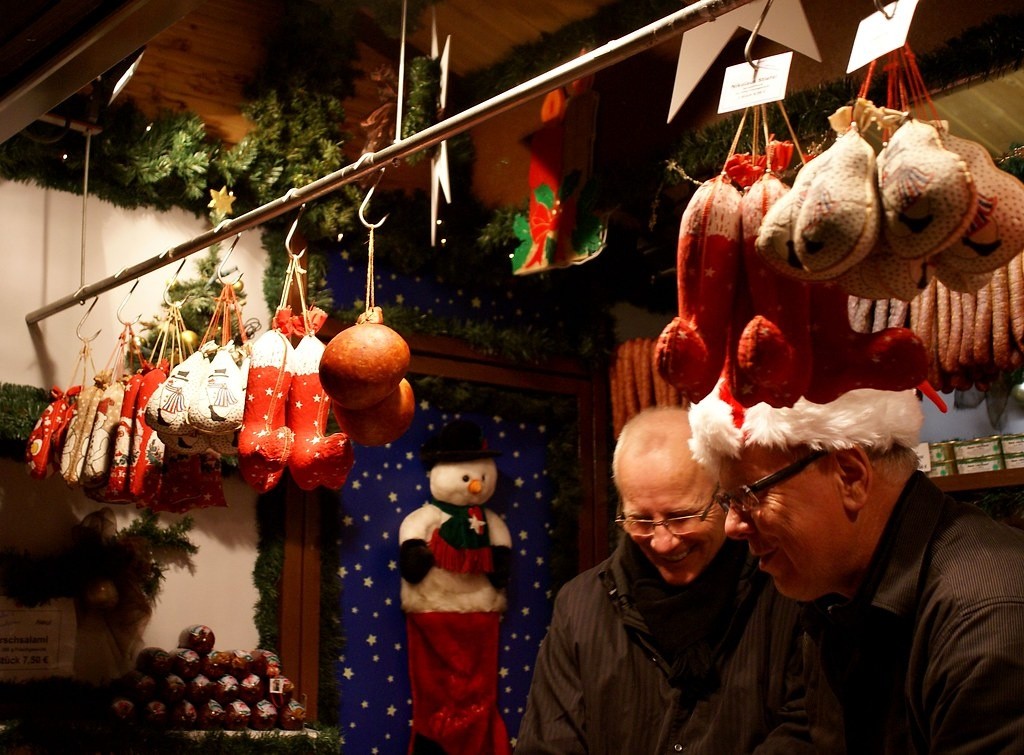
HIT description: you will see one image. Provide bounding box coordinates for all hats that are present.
[688,351,924,468]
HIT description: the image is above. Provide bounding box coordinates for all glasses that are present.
[614,486,721,537]
[714,446,828,514]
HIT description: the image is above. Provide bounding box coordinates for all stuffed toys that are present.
[396,427,514,755]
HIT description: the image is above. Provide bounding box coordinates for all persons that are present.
[688,365,1024,755]
[513,406,852,754]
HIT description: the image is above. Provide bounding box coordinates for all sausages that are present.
[108,626,307,732]
[607,335,697,441]
[846,251,1024,401]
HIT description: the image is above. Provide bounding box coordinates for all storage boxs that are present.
[0,595,154,682]
